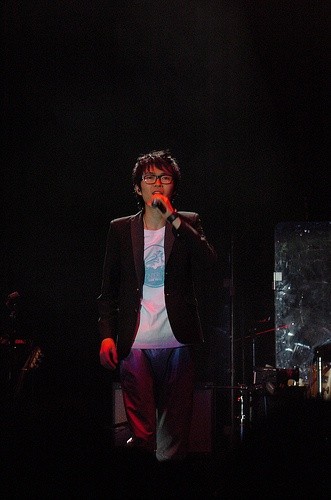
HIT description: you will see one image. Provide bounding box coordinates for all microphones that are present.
[152,199,161,208]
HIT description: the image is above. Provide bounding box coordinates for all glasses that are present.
[140,175,173,184]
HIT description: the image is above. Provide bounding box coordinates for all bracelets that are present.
[166,211,179,224]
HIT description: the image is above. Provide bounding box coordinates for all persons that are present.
[97,150,218,500]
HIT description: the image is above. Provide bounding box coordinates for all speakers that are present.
[112,382,218,459]
